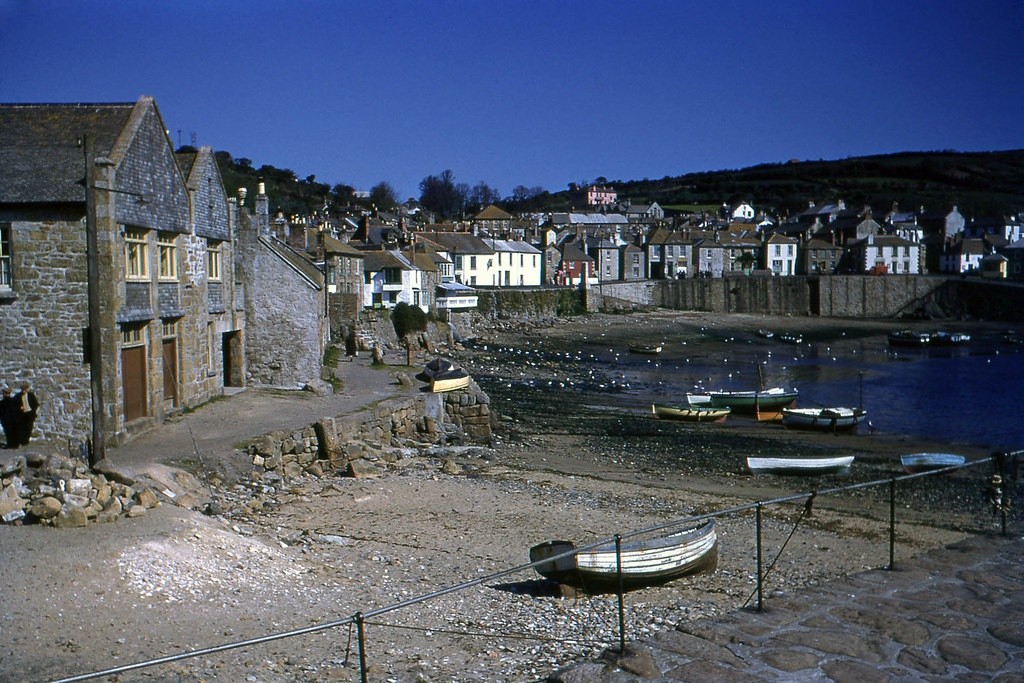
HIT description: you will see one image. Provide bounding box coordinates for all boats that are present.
[530,519,720,587]
[650,400,731,424]
[744,453,858,477]
[884,328,976,349]
[686,385,800,414]
[901,453,971,475]
[628,341,663,354]
[428,367,471,394]
[781,408,870,428]
[423,358,453,378]
[756,327,774,338]
[779,335,804,345]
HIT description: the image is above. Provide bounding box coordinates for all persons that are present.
[13,382,40,446]
[344,335,359,362]
[0,388,21,449]
